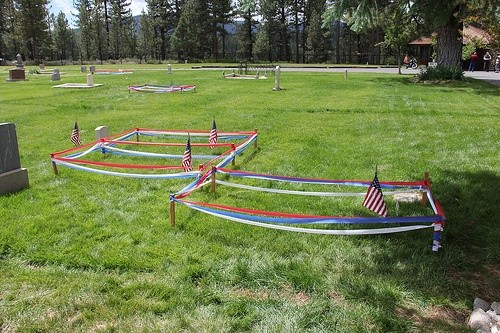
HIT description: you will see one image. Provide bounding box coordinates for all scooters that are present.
[403,55,419,69]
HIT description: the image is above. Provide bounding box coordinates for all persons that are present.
[403,51,410,69]
[468,51,478,72]
[493,50,500,73]
[483,50,492,72]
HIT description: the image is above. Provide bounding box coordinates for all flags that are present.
[69,121,81,146]
[181,136,192,173]
[362,171,388,218]
[208,119,218,150]
